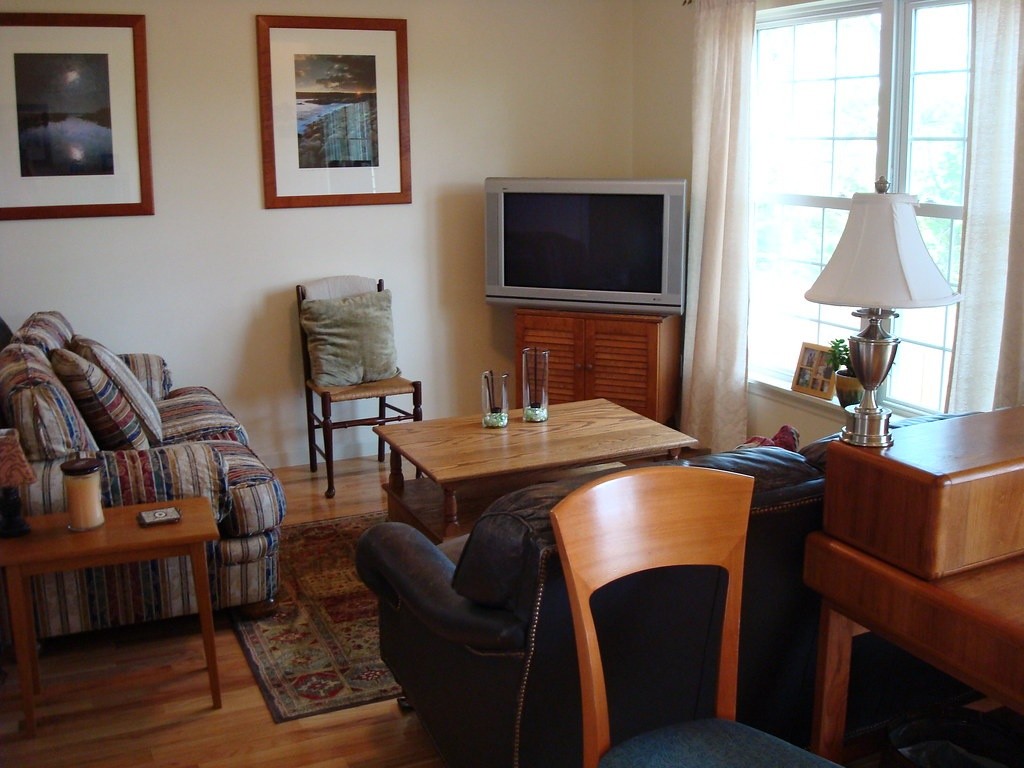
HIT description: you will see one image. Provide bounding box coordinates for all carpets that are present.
[226,508,410,724]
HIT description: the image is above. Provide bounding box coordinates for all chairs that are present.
[296,274,422,498]
[549,465,849,768]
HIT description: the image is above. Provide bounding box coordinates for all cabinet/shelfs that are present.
[803,405,1024,763]
[515,308,683,429]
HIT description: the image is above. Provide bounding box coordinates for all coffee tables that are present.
[373,397,699,548]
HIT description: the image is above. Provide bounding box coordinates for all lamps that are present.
[0,428,35,539]
[804,176,960,448]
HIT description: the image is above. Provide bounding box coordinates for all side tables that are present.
[0,497,222,738]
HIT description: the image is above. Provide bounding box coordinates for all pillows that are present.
[300,289,402,388]
[51,346,152,452]
[70,334,163,444]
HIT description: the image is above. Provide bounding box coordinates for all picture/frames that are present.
[791,341,839,401]
[0,12,156,222]
[254,14,412,210]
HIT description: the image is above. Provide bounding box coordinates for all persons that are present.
[806,353,817,367]
[823,364,832,379]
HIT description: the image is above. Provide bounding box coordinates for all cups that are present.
[482,370,508,427]
[522,346,549,422]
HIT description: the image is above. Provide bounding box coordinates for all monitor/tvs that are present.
[484,177,687,316]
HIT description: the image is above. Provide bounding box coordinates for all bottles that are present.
[60,458,105,530]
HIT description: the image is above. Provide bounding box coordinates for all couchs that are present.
[0,310,286,645]
[354,411,986,768]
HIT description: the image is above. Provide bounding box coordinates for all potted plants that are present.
[826,338,866,409]
